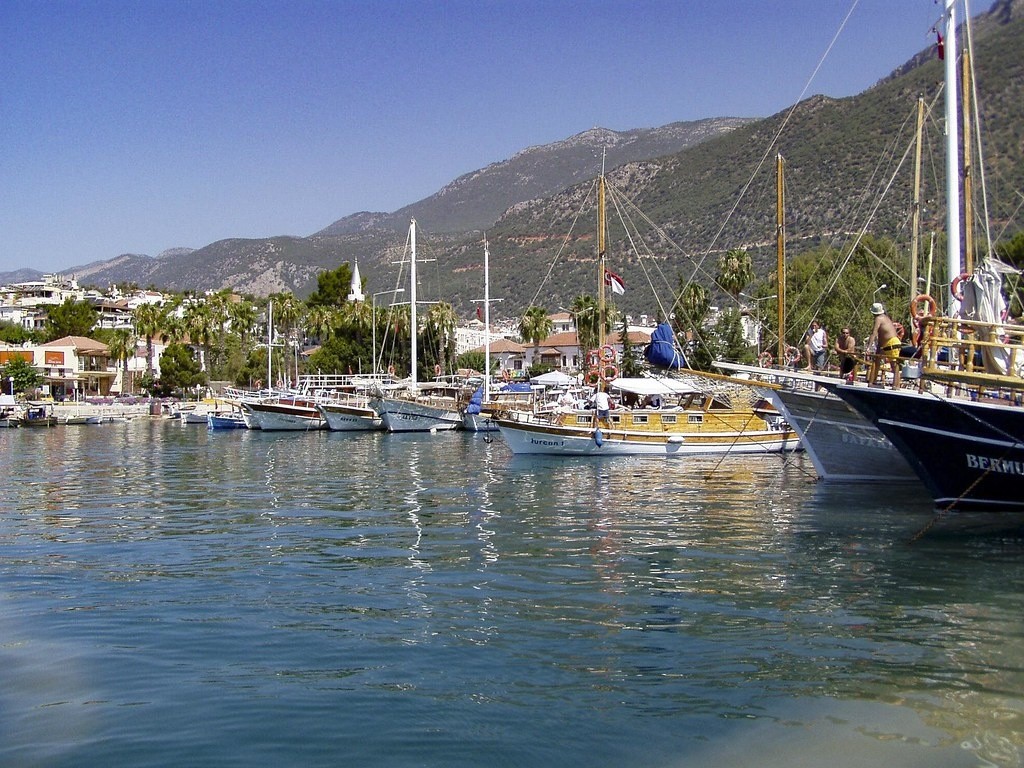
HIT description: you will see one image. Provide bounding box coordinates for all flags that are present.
[611,277,625,296]
[604,268,624,290]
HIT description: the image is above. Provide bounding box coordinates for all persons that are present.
[865,303,902,390]
[588,387,663,428]
[555,389,573,425]
[834,327,857,379]
[804,320,829,372]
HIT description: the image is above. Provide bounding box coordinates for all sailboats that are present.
[0,0,1024,519]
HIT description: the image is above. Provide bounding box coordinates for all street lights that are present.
[739,292,778,357]
[872,283,887,303]
[371,288,406,374]
[917,276,948,317]
[559,306,594,369]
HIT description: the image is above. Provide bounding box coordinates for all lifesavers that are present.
[586,350,602,368]
[502,370,508,380]
[893,318,940,339]
[388,366,395,376]
[586,371,601,388]
[953,309,977,334]
[951,273,972,302]
[994,301,1011,345]
[599,344,616,362]
[785,346,800,363]
[758,351,772,366]
[911,294,936,318]
[435,365,441,375]
[599,364,618,382]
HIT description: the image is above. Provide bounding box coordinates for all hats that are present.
[869,303,884,315]
[563,386,568,390]
